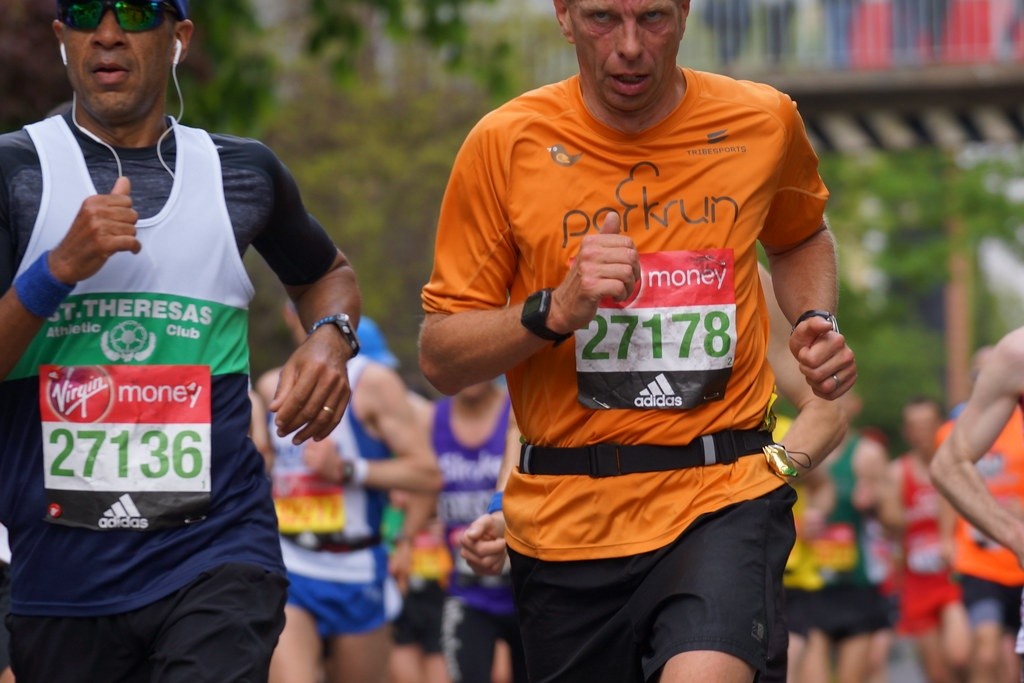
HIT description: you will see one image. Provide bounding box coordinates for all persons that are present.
[419,0,859,683]
[248,301,523,683]
[0,0,360,683]
[459,261,1024,683]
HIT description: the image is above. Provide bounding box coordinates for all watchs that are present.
[521,288,575,348]
[790,310,838,334]
[309,313,360,358]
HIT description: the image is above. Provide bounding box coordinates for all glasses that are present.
[57,0,176,33]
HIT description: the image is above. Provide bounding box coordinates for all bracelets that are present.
[488,492,504,513]
[15,251,75,318]
[354,459,367,484]
[339,458,352,484]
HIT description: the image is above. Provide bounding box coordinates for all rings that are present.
[832,376,840,386]
[323,406,334,414]
